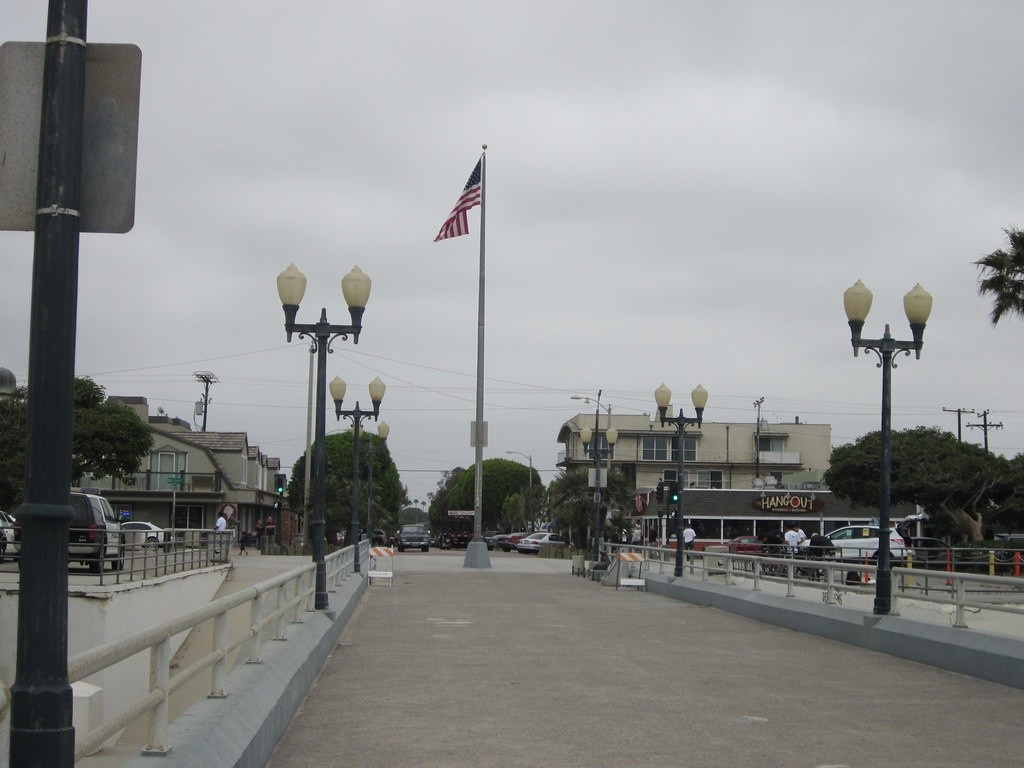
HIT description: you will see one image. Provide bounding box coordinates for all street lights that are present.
[577,408,619,559]
[841,278,935,614]
[571,396,616,468]
[350,420,391,546]
[507,451,534,490]
[325,372,386,573]
[275,262,374,614]
[654,381,709,575]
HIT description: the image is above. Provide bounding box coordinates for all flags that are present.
[434,158,482,243]
[636,495,643,512]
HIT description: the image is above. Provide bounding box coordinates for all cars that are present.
[517,532,575,554]
[702,535,792,555]
[0,512,22,554]
[334,526,388,547]
[910,536,958,560]
[119,521,174,550]
[485,529,531,554]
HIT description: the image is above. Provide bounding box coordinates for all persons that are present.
[648,525,656,559]
[621,523,641,551]
[214,511,226,530]
[684,524,697,560]
[266,517,276,544]
[238,531,248,555]
[255,520,265,550]
[759,523,834,575]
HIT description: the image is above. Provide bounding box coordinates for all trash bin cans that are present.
[705,546,730,576]
[185,532,200,547]
[572,555,585,577]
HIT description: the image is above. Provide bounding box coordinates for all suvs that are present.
[797,524,908,566]
[396,524,430,550]
[13,485,138,572]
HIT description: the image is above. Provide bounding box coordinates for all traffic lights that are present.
[277,477,283,496]
[670,483,680,505]
[669,509,679,519]
[655,483,664,504]
